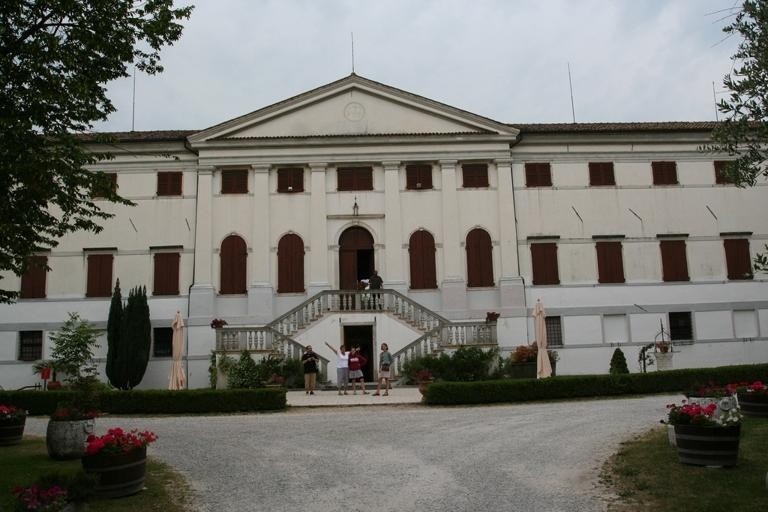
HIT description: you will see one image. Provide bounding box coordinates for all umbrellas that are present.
[168,310,186,390]
[532,298,552,379]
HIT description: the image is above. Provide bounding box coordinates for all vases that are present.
[417,380,433,401]
[91,447,147,497]
[0,418,26,444]
[47,417,96,461]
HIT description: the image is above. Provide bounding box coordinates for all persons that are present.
[301,345,319,395]
[371,342,394,396]
[370,270,383,310]
[348,347,369,394]
[324,341,360,395]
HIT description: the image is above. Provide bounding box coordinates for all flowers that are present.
[660,379,768,467]
[84,426,159,452]
[48,402,98,422]
[416,369,434,380]
[0,405,30,417]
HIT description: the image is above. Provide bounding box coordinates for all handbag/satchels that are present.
[382,364,389,371]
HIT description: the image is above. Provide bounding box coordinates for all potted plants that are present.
[29,358,55,379]
[45,360,67,390]
